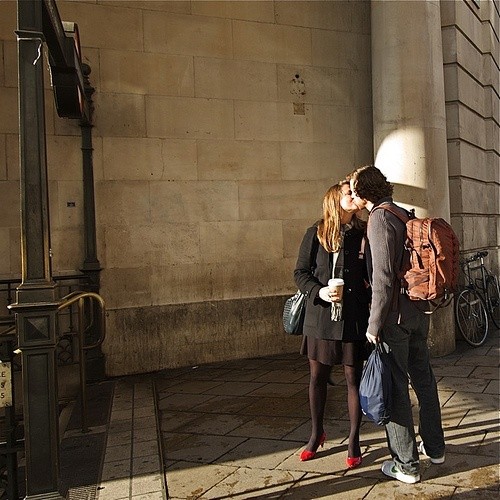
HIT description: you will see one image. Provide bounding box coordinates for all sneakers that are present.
[382,460,420,484]
[420,441,446,463]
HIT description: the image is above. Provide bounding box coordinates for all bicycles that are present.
[455,250,500,348]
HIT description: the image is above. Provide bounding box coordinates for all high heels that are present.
[301,431,326,461]
[346,456,362,468]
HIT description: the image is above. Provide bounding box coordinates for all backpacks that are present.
[371,204,460,301]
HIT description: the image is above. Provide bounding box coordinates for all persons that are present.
[293,180,369,468]
[345,166,445,483]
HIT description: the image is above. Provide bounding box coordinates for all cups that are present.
[328,279,344,301]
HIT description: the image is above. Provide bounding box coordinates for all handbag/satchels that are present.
[359,339,391,425]
[283,291,305,334]
